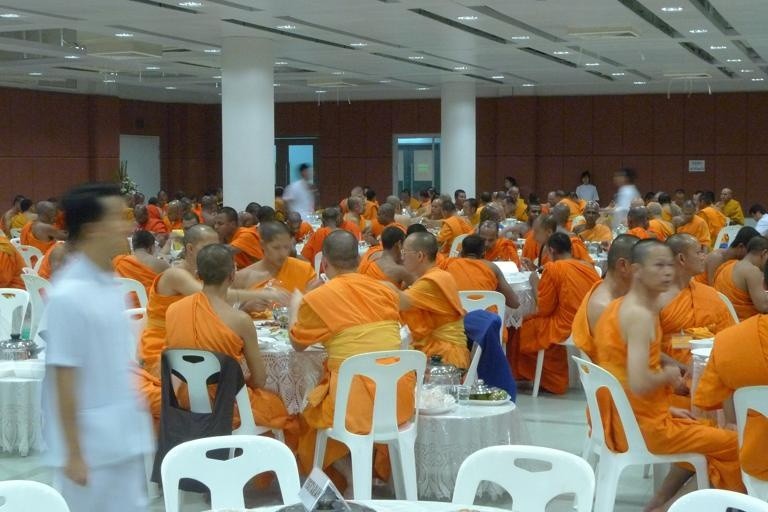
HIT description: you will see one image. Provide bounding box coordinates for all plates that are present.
[156,249,185,265]
[414,352,511,415]
[246,305,325,349]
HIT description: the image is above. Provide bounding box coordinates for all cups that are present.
[299,208,628,259]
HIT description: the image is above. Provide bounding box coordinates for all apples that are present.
[469,390,507,400]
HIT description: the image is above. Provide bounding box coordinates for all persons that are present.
[280,164,319,223]
[503,174,520,199]
[0,193,68,302]
[112,187,767,512]
[574,169,601,206]
[609,168,642,221]
[36,182,156,512]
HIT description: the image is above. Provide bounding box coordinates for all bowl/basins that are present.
[688,336,715,365]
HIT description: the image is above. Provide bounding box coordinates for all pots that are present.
[0,330,48,363]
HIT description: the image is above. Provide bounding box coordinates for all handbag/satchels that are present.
[151,347,245,492]
